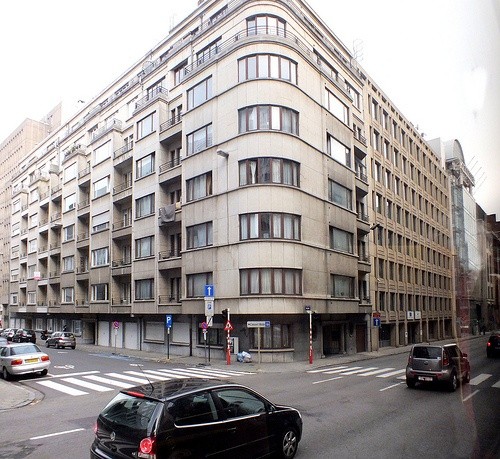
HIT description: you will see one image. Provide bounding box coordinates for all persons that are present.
[479,317,486,335]
[62,325,67,332]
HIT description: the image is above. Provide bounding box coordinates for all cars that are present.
[45,331,76,349]
[486,332,500,357]
[89,377,304,459]
[12,328,37,343]
[405,341,471,391]
[0,342,51,380]
[0,328,18,341]
